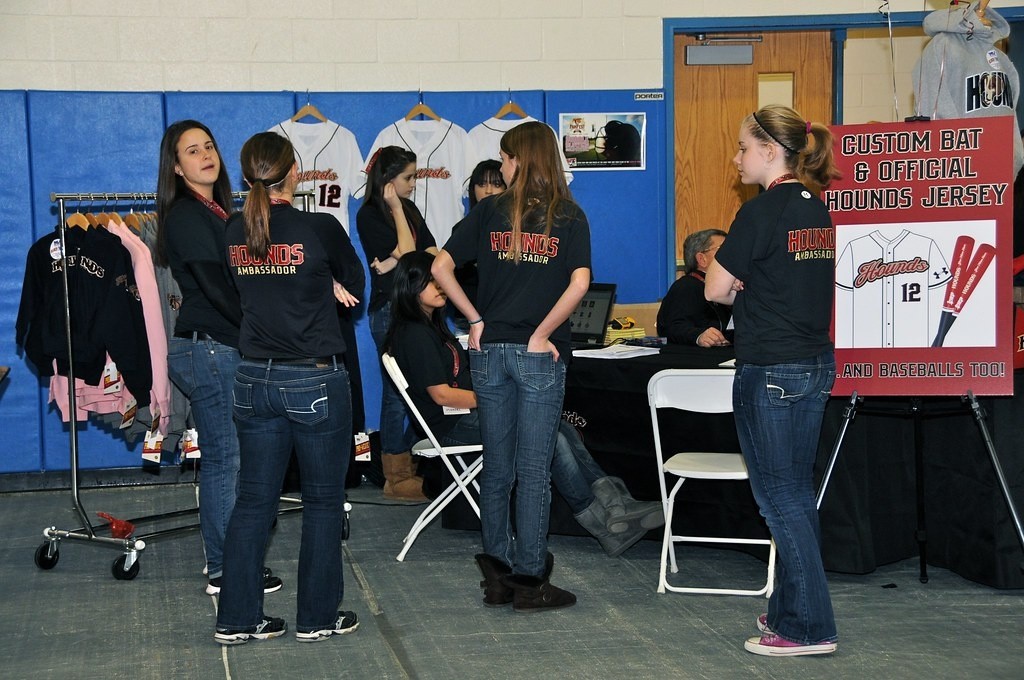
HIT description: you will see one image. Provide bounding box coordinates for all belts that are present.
[174,330,213,340]
[263,357,335,366]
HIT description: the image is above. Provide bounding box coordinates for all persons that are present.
[155,121,284,596]
[430,121,593,614]
[914,0,1024,258]
[705,106,839,656]
[657,229,735,347]
[383,252,666,558]
[452,159,507,335]
[356,147,439,501]
[214,132,360,645]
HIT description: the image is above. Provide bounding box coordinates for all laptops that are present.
[571,282,616,349]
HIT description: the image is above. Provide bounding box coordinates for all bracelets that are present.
[468,318,483,324]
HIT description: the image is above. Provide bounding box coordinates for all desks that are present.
[560,352,773,563]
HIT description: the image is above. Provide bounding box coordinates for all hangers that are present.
[66,191,243,234]
[495,87,527,119]
[289,89,327,124]
[406,88,441,123]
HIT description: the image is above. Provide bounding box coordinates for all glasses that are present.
[702,245,720,254]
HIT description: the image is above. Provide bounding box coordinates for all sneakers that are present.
[744,633,839,656]
[756,613,775,634]
[203,565,272,577]
[296,611,360,642]
[214,616,286,645]
[206,572,282,596]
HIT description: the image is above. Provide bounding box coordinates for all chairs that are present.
[383,351,484,560]
[645,369,778,600]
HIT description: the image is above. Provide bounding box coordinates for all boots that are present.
[573,498,647,558]
[474,554,511,608]
[505,554,575,613]
[380,450,428,501]
[592,475,665,534]
